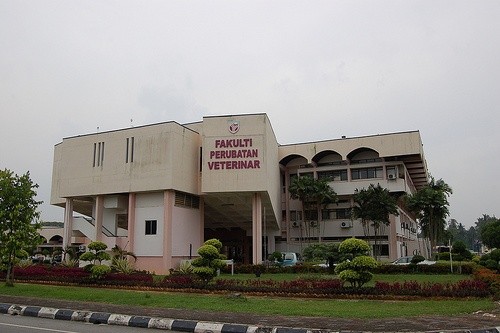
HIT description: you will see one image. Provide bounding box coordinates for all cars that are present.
[389,255,438,265]
[266,252,304,268]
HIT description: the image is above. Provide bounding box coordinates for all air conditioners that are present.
[388,173,395,179]
[340,220,352,228]
[292,221,301,227]
[310,220,317,226]
